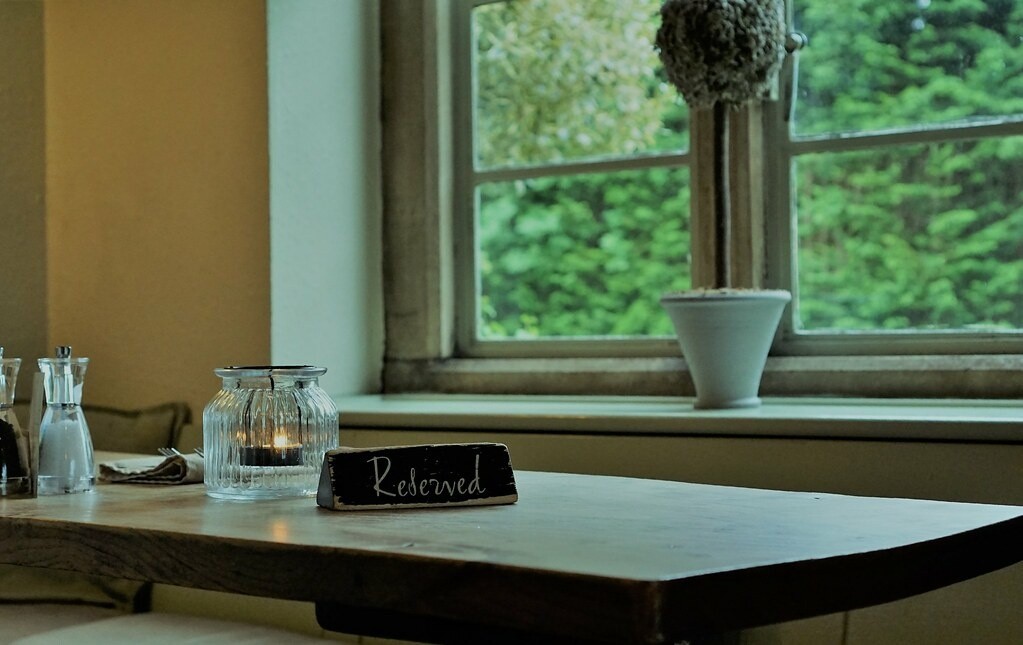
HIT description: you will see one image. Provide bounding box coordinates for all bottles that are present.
[37,346,96,496]
[203,364,339,500]
[0,346,37,499]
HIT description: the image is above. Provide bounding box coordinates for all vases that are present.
[659,289,792,409]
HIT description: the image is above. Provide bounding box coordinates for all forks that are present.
[157,446,183,459]
[194,447,203,458]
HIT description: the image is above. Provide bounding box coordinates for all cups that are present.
[658,289,791,409]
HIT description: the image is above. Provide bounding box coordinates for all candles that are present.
[239,430,303,466]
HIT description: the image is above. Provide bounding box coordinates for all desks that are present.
[1,447,1023,645]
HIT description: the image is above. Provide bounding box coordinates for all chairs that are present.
[0,400,194,614]
